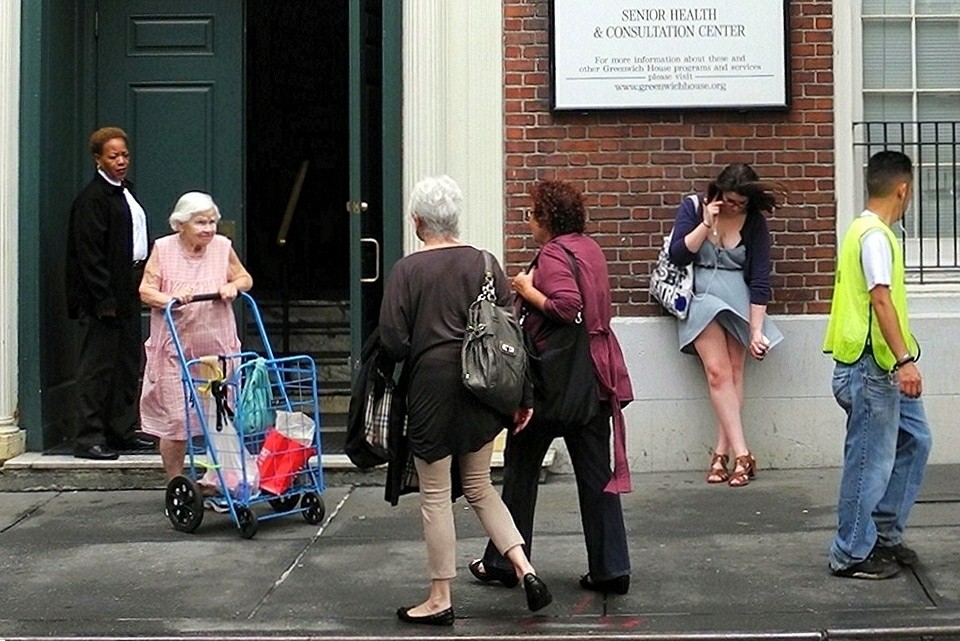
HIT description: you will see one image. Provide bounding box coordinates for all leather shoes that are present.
[74,443,119,460]
[128,438,156,448]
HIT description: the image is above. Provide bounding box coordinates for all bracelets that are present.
[897,354,914,367]
[704,220,711,228]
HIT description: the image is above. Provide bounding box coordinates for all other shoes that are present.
[202,493,229,513]
[396,605,454,628]
[523,573,554,613]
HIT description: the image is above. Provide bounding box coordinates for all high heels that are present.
[728,450,756,486]
[706,452,729,482]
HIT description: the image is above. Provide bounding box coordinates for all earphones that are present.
[713,228,718,236]
[901,192,906,202]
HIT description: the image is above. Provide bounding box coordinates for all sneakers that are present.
[872,544,918,565]
[829,554,901,579]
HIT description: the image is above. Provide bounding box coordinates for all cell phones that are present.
[714,190,723,202]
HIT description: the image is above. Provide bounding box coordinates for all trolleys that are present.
[165,289,326,540]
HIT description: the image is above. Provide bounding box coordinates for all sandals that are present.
[580,571,630,596]
[469,558,519,590]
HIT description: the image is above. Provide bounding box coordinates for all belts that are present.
[863,344,873,355]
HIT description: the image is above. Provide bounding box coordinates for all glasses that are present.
[721,193,749,209]
[528,210,538,216]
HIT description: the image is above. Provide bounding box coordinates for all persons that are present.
[469,180,634,594]
[66,127,156,460]
[822,151,933,579]
[668,164,789,486]
[380,177,552,625]
[138,192,253,512]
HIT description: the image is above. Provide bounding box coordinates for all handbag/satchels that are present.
[461,249,527,415]
[198,354,317,503]
[511,241,602,432]
[650,194,700,320]
